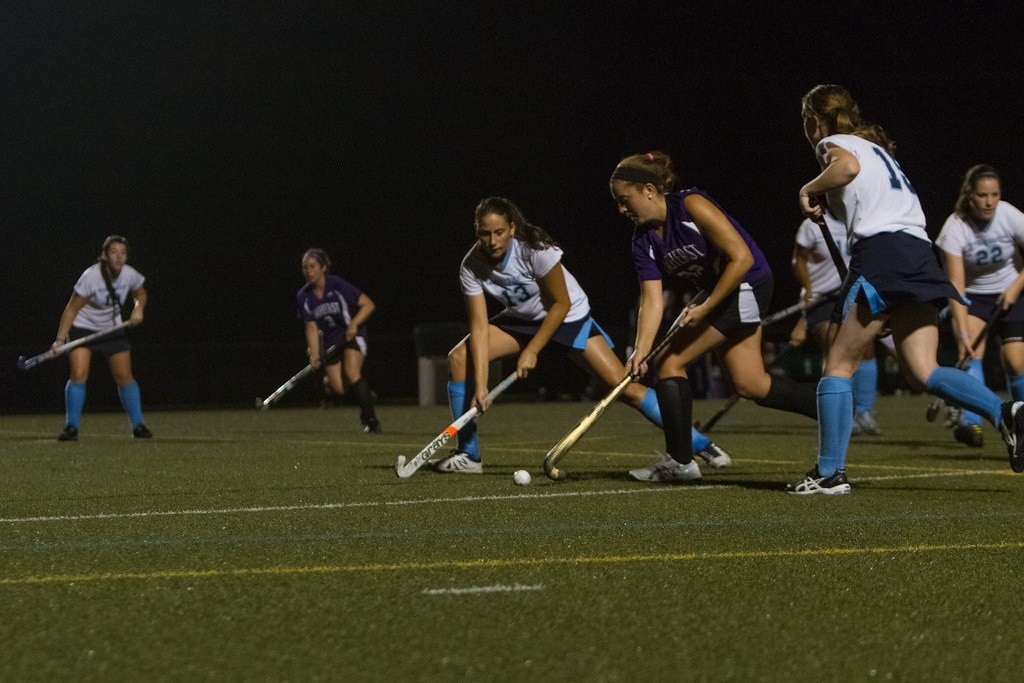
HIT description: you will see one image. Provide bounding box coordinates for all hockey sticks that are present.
[541,317,690,483]
[760,297,817,326]
[394,371,519,478]
[925,304,1004,423]
[692,340,797,434]
[255,338,348,411]
[17,319,130,372]
[808,195,891,339]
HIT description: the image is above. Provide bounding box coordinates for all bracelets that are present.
[57,337,65,343]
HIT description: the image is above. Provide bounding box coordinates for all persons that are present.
[935,163,1024,449]
[300,248,382,433]
[789,193,898,435]
[786,85,1024,494]
[610,153,857,481]
[49,235,153,441]
[428,196,731,473]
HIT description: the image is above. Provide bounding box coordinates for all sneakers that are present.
[628,453,702,482]
[786,462,851,495]
[698,442,731,467]
[133,424,152,439]
[427,448,483,473]
[945,405,959,427]
[995,399,1024,473]
[58,424,78,442]
[856,408,882,435]
[850,417,862,435]
[954,422,985,450]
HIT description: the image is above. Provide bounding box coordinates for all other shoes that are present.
[361,412,381,431]
[323,377,337,400]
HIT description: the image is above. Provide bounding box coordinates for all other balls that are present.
[513,469,531,486]
